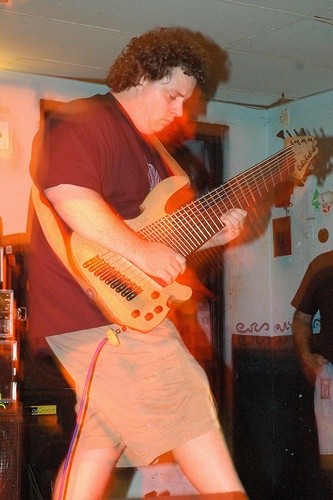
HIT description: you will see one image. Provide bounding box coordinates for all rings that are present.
[235,226,240,230]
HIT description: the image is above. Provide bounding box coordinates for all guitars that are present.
[65,127,320,334]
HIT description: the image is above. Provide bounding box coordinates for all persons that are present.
[25,26,249,500]
[291,250,333,500]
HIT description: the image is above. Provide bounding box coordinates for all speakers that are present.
[20,332,77,393]
[24,392,77,500]
[0,408,24,500]
